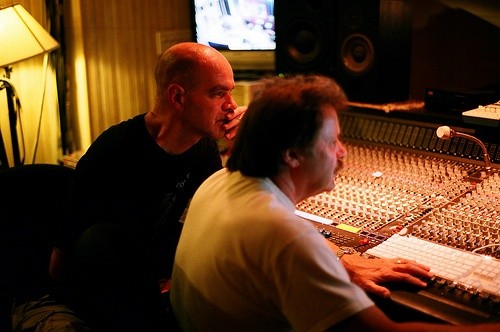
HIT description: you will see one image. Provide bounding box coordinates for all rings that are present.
[398,259,400,264]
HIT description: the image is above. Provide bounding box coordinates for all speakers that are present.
[275,0,412,103]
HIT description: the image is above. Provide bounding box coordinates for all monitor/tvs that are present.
[188,0,276,72]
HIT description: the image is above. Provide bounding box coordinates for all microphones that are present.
[435,126,491,170]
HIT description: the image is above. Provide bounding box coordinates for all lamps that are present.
[0,3,60,167]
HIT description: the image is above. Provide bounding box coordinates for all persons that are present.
[169,75,500,332]
[49,41,238,332]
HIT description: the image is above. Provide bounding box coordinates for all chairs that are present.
[0,163,75,332]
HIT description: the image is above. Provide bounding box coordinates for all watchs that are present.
[337,246,355,261]
